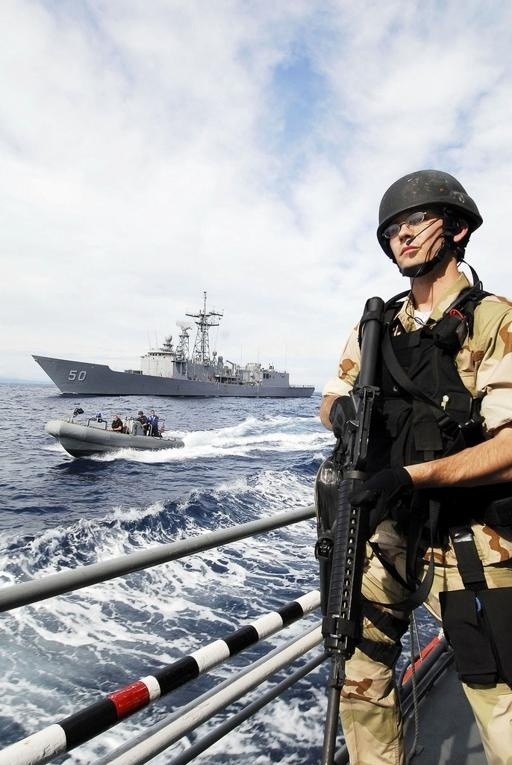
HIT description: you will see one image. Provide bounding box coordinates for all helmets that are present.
[377,170,483,260]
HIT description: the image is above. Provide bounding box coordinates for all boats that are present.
[31,291,315,399]
[45,408,184,458]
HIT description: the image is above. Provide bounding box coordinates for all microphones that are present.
[405,215,441,247]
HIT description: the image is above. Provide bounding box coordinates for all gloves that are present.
[349,467,415,542]
[329,396,357,437]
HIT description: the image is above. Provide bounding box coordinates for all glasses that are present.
[382,212,427,239]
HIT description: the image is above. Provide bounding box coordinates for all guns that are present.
[315,296,385,765]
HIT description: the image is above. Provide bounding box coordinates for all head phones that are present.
[441,212,465,239]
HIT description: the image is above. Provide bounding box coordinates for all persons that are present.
[148,409,159,437]
[137,411,149,435]
[318,167,512,765]
[107,415,123,433]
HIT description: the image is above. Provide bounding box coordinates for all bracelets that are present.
[113,428,114,430]
